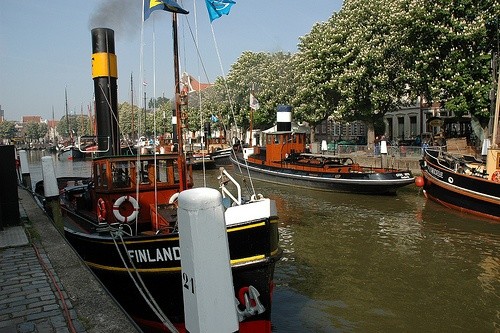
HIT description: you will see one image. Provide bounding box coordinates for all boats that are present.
[18,133,229,163]
[230,79,417,196]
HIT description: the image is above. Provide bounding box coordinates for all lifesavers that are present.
[96,198,106,221]
[113,195,140,223]
[491,171,500,182]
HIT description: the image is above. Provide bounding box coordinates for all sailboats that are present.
[35,1,283,333]
[418,50,499,220]
